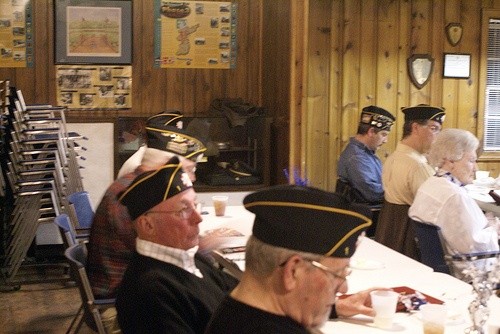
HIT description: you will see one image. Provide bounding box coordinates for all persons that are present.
[204,182,373,334]
[338,106,395,238]
[116,108,183,178]
[85,123,208,301]
[115,156,394,334]
[407,129,500,295]
[374,103,446,262]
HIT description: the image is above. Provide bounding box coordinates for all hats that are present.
[243,186,373,258]
[145,127,207,163]
[115,156,193,221]
[361,105,396,131]
[401,104,446,122]
[144,111,184,130]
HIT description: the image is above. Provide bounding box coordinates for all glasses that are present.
[301,257,353,284]
[144,202,201,221]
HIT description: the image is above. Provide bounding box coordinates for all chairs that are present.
[332,178,500,288]
[0,79,120,334]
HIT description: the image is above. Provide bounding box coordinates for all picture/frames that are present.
[53,0,134,65]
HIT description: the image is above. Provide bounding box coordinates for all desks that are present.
[464,180,500,216]
[186,189,500,334]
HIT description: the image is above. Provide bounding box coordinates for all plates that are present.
[472,179,495,186]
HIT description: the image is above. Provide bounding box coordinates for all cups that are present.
[476,170,489,182]
[212,196,229,216]
[419,305,448,334]
[369,290,400,330]
